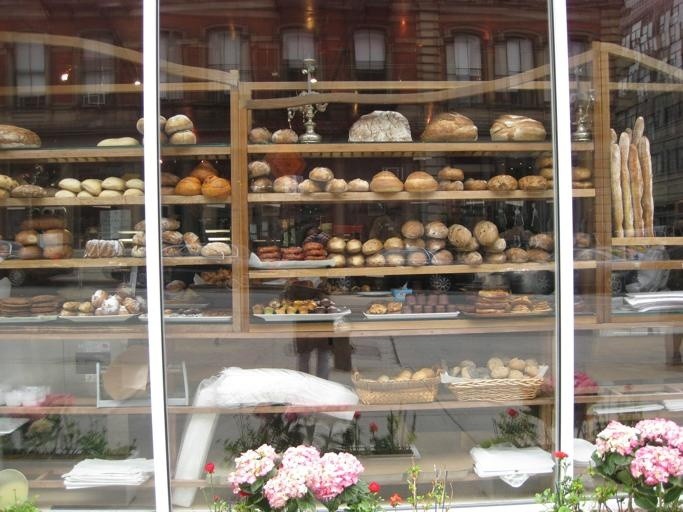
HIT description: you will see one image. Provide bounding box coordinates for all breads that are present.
[447,357,540,380]
[247,126,270,142]
[490,114,547,143]
[327,220,595,268]
[83,217,230,259]
[12,215,74,260]
[248,160,273,193]
[439,167,464,191]
[348,179,369,191]
[249,297,342,316]
[536,157,553,189]
[373,367,437,380]
[487,175,518,190]
[139,308,232,318]
[458,290,553,315]
[95,136,139,148]
[464,178,488,190]
[272,174,297,193]
[347,109,414,143]
[0,173,60,200]
[366,294,456,316]
[611,117,654,238]
[404,171,439,192]
[572,166,592,189]
[54,170,144,198]
[518,175,547,190]
[135,115,197,146]
[1,295,58,317]
[297,167,348,193]
[370,171,404,192]
[421,112,479,142]
[271,129,300,145]
[257,236,326,263]
[161,166,231,198]
[61,283,141,316]
[1,125,42,151]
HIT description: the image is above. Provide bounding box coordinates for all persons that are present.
[280,277,334,379]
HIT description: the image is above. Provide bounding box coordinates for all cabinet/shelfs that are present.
[1,30,238,488]
[604,42,682,434]
[238,41,604,491]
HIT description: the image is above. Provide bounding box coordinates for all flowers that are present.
[205,443,379,512]
[224,404,417,454]
[536,450,585,512]
[592,418,682,511]
[481,408,539,449]
[2,386,96,456]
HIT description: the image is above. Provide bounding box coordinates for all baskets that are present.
[444,367,543,401]
[351,365,441,405]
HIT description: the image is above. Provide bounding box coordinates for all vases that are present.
[333,446,421,484]
[1,453,139,507]
[470,442,557,479]
